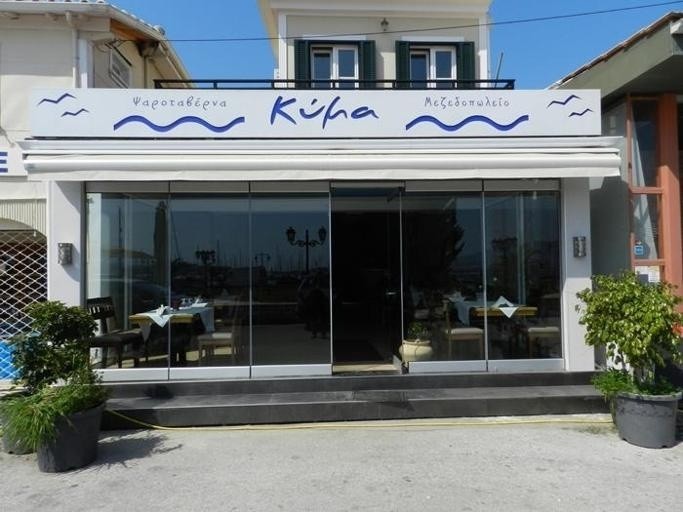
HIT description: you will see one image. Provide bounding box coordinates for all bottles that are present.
[634,239,647,260]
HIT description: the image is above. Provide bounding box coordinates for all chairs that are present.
[426,299,484,360]
[197,293,244,365]
[515,293,562,357]
[87,297,148,367]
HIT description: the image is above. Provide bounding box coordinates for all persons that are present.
[295,269,329,338]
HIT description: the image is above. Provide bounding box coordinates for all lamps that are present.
[380,18,389,31]
[574,236,587,258]
[58,243,72,264]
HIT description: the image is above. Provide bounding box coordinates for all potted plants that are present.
[399,322,434,367]
[0,300,114,473]
[575,271,683,448]
